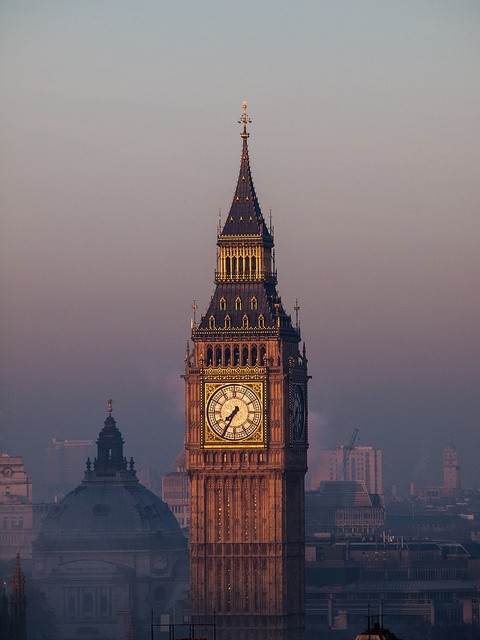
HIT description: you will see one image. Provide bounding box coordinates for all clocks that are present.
[286,351,310,451]
[199,356,271,452]
